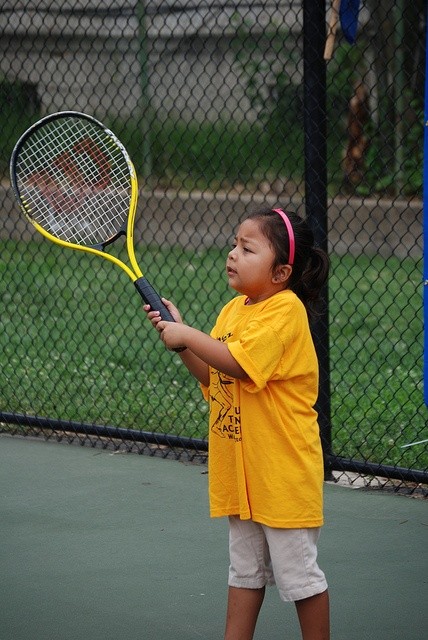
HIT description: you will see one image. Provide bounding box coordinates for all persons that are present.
[141,208,331,640]
